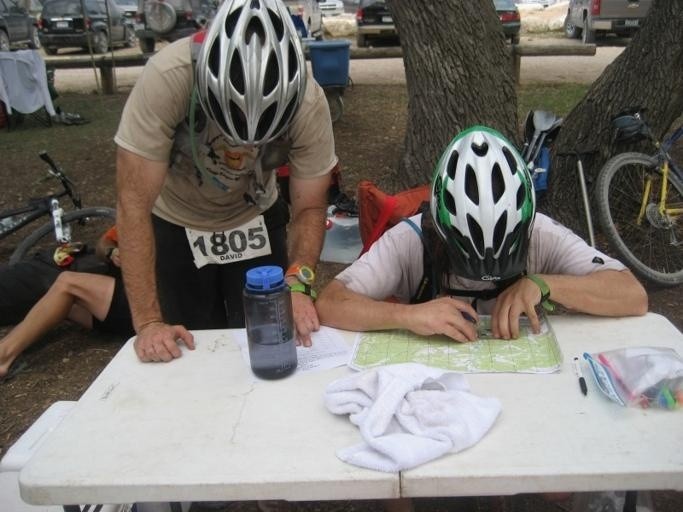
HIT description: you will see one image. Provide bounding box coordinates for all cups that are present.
[243,265,298,380]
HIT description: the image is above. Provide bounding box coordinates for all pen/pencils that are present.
[461,311,477,322]
[574,357,586,394]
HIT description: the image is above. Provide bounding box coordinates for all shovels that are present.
[521,109,563,171]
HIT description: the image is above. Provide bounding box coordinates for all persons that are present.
[0,266,136,381]
[1,224,120,328]
[112,0,340,363]
[313,125,649,343]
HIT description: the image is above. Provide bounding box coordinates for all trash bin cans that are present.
[308,40,350,87]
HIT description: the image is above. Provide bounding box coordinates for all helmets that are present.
[430,126,537,282]
[194,0,307,148]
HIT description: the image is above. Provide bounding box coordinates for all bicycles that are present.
[596,106,682,288]
[0,150,119,268]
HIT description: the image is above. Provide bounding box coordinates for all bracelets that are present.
[104,247,114,260]
[290,284,317,302]
[521,274,555,312]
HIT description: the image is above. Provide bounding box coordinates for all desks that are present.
[400,311,682,511]
[18,312,400,512]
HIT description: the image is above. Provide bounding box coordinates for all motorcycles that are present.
[320,85,344,123]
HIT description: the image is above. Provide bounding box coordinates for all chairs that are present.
[0,49,56,132]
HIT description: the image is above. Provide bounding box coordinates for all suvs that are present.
[136,0,218,54]
[38,0,137,55]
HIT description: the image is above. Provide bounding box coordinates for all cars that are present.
[493,0,521,44]
[283,0,322,41]
[564,0,653,46]
[356,0,401,46]
[0,0,43,52]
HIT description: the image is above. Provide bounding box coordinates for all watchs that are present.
[285,264,315,286]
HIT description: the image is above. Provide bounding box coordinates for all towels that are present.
[324,361,502,473]
